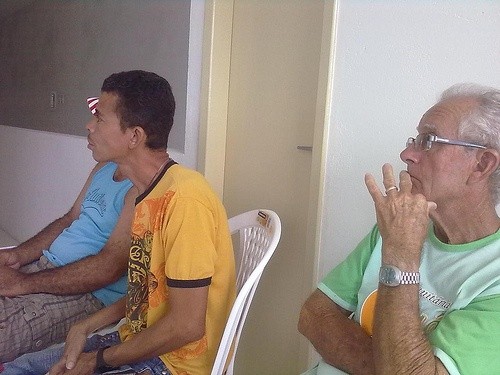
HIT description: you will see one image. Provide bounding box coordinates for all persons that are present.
[0,138,168,362]
[0,69,237,375]
[297,82,500,375]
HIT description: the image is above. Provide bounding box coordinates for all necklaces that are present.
[145,158,170,191]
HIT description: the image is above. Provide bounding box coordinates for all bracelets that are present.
[96,345,119,372]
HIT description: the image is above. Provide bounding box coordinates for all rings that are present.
[385,186,398,194]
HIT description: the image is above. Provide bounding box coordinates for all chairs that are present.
[210,208,282,375]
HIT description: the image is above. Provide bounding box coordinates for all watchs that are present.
[379,264,420,287]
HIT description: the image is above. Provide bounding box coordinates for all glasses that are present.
[405,133,488,152]
